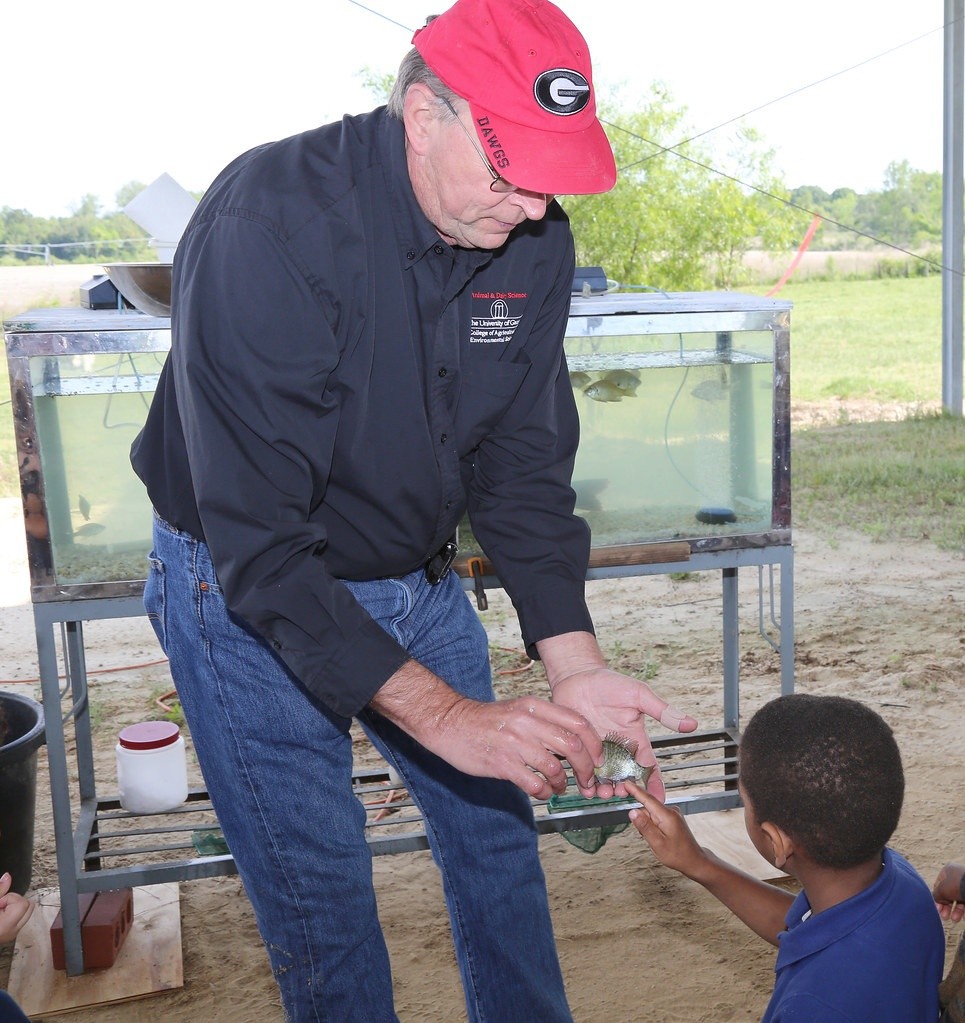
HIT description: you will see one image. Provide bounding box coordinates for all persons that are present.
[0,873,35,1023]
[625,694,946,1023]
[933,864,965,922]
[130,0,697,1023]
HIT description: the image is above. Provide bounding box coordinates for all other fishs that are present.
[591,732,656,790]
[569,478,609,510]
[691,381,733,401]
[61,522,106,539]
[569,372,592,388]
[598,368,642,391]
[584,380,638,403]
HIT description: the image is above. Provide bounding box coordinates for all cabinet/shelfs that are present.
[29,547,797,978]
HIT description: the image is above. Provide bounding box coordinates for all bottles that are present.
[115,721,188,815]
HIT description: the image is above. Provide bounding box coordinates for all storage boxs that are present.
[0,294,795,598]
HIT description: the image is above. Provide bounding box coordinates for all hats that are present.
[409,1,618,196]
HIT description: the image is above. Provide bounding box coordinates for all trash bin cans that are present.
[0,691,48,897]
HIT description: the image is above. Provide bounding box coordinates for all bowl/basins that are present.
[101,263,173,318]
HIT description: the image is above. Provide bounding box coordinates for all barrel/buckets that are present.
[0,690,47,896]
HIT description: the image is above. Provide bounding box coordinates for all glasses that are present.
[436,94,519,192]
